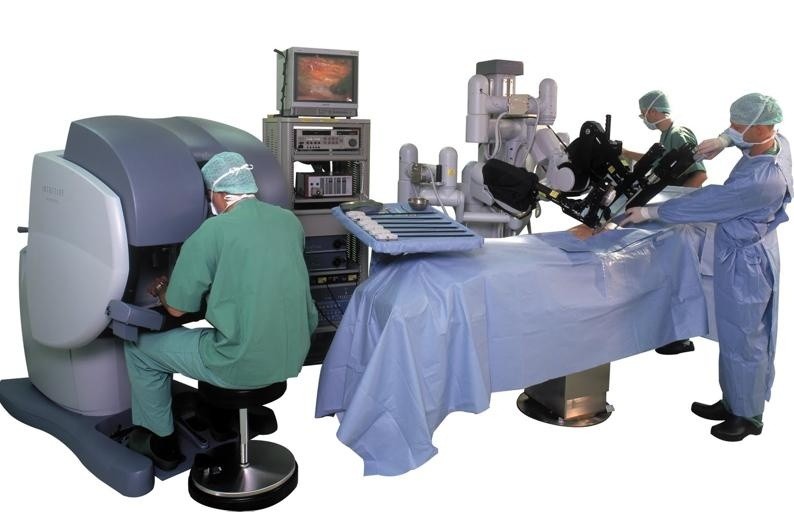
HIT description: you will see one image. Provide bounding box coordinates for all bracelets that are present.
[154,282,167,295]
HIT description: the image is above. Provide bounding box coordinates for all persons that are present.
[620,87,707,356]
[109,150,320,473]
[618,92,794,440]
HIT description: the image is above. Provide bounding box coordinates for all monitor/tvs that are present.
[276,46,360,117]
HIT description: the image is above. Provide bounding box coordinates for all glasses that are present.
[638,113,646,120]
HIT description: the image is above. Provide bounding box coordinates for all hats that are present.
[201,152,258,195]
[639,89,671,115]
[730,93,784,127]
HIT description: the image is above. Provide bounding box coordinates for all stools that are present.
[188,380,298,511]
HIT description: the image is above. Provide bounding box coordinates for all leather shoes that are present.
[712,416,763,442]
[691,400,728,421]
[656,341,695,355]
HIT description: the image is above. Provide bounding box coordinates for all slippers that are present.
[121,432,176,471]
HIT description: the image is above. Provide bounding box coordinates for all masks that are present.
[728,125,754,150]
[209,189,224,216]
[643,108,660,130]
